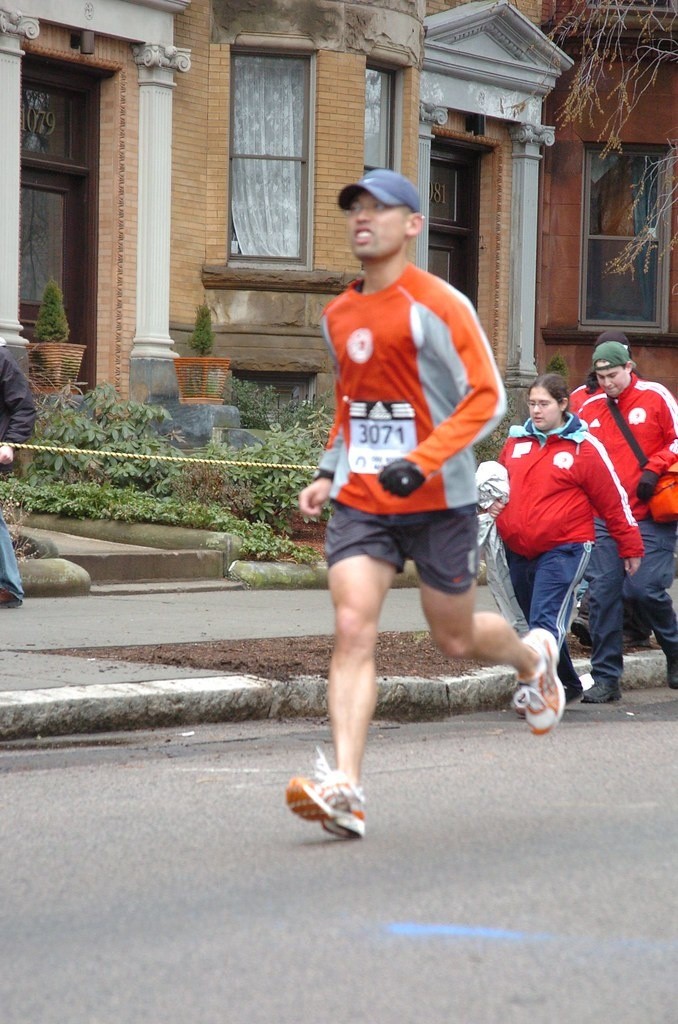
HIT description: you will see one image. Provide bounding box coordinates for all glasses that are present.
[526,399,562,409]
[342,202,410,216]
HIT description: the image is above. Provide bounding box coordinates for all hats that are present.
[592,340,637,370]
[594,329,632,354]
[337,169,420,213]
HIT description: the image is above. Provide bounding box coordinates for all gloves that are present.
[639,471,656,500]
[378,460,425,498]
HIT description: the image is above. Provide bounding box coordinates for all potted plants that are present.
[173,297,231,406]
[26,276,87,396]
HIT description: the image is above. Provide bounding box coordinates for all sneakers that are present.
[667,656,678,689]
[515,627,565,735]
[287,746,365,840]
[582,681,621,704]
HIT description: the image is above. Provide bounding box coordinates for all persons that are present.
[478,373,645,717]
[287,169,567,837]
[580,340,678,704]
[0,336,37,609]
[568,330,638,414]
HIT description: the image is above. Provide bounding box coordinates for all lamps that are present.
[70,28,95,55]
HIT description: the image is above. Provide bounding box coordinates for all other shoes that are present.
[0,589,21,609]
[570,618,592,646]
